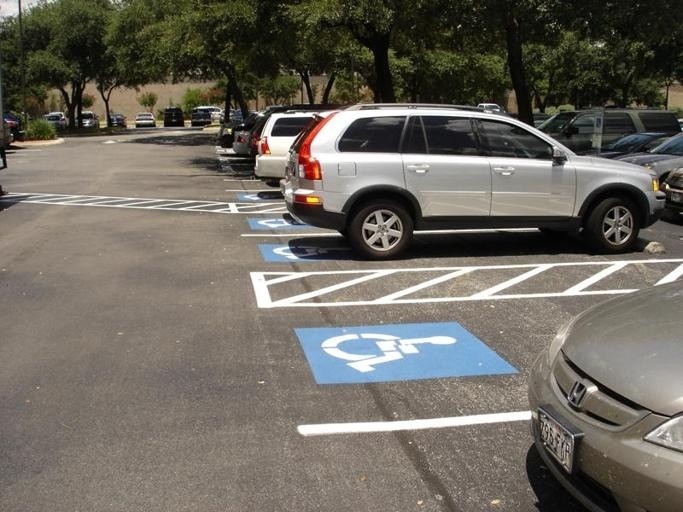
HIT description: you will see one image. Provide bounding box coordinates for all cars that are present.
[528,280,683,512]
